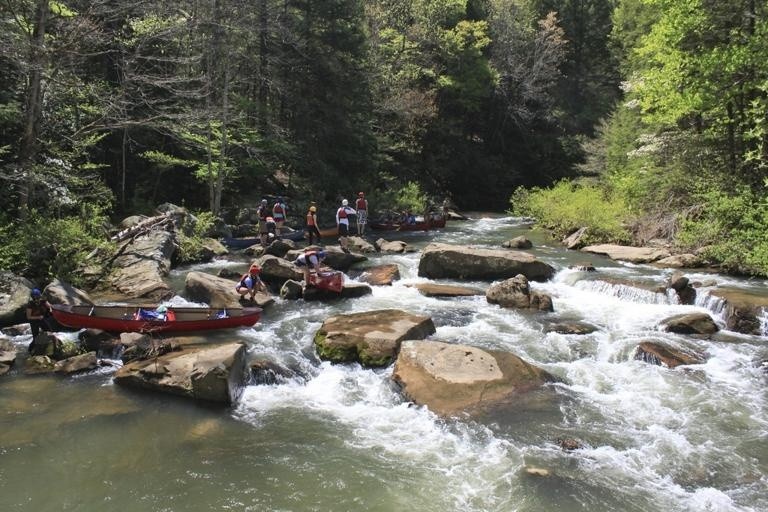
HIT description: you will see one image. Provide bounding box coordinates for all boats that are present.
[223,226,337,247]
[45,301,264,332]
[309,270,345,293]
[379,218,446,231]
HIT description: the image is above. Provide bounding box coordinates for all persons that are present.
[336,199,357,237]
[271,198,287,236]
[234,264,268,304]
[27,289,56,353]
[292,250,327,291]
[355,191,369,238]
[257,199,269,248]
[306,205,322,246]
[397,195,451,228]
[265,212,280,246]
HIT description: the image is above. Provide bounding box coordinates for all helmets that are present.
[319,251,326,260]
[259,192,365,213]
[250,264,258,274]
